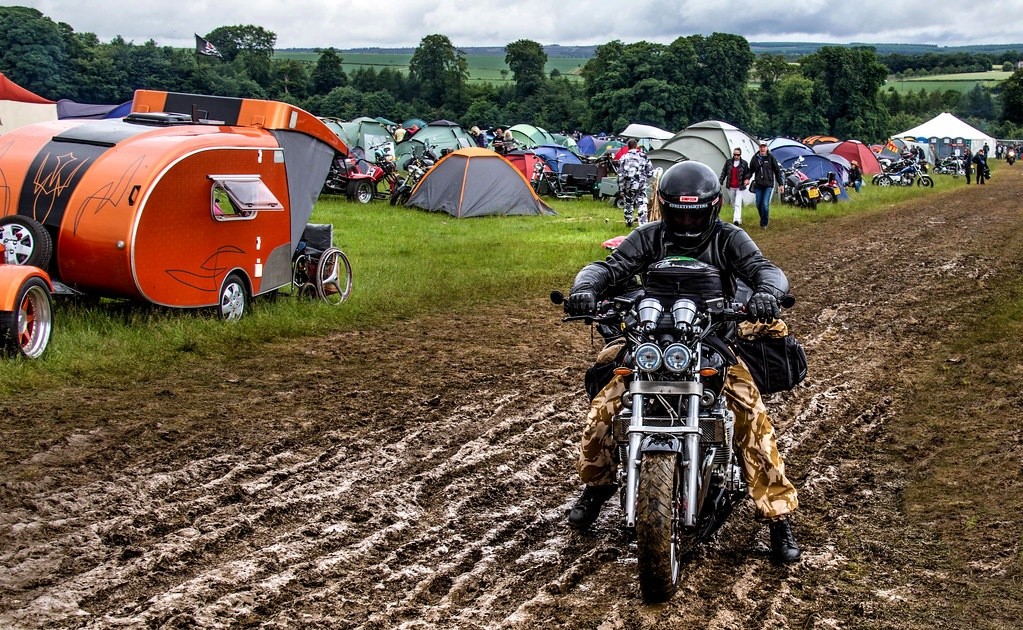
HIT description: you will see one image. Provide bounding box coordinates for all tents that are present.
[318,113,995,219]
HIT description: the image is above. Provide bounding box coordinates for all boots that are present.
[568,481,618,529]
[769,518,801,563]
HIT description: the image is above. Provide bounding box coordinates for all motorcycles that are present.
[528,151,624,209]
[938,153,976,176]
[545,255,783,603]
[779,155,840,208]
[390,139,453,207]
[1006,149,1015,165]
[328,143,406,205]
[872,147,934,191]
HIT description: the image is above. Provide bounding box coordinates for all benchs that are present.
[560,162,598,186]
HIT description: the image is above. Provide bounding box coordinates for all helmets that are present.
[656,160,722,256]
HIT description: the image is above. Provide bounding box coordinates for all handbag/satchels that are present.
[584,318,808,403]
[749,180,756,193]
[980,160,985,164]
[644,257,723,314]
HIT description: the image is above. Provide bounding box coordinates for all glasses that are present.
[733,154,740,156]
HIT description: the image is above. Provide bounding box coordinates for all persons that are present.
[395,123,406,143]
[745,141,784,228]
[618,139,653,227]
[997,144,1023,160]
[504,130,520,153]
[901,146,907,154]
[720,147,748,225]
[964,149,973,184]
[842,160,862,193]
[910,145,918,157]
[561,161,802,565]
[983,142,989,158]
[493,128,506,155]
[403,124,420,141]
[916,146,925,160]
[973,150,986,185]
[470,125,489,149]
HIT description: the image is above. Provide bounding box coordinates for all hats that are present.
[759,141,767,147]
[850,160,860,167]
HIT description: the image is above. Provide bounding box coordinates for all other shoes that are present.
[734,221,739,226]
[627,223,632,228]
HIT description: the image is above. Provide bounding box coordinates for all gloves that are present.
[566,290,597,325]
[747,292,780,325]
